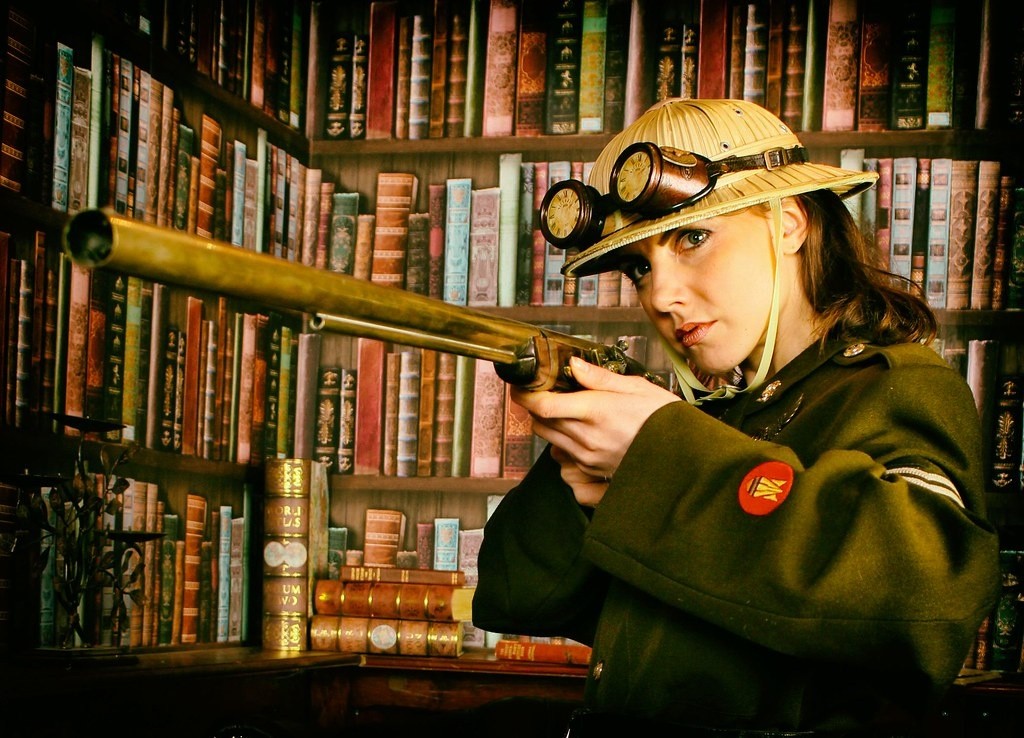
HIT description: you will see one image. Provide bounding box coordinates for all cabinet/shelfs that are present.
[0,0,1024,738]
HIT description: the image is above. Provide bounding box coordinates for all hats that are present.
[559,97,877,280]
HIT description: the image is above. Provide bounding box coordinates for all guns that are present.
[63,207,670,399]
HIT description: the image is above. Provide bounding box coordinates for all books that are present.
[0,0,1024,667]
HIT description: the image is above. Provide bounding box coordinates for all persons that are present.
[472,97,1003,738]
